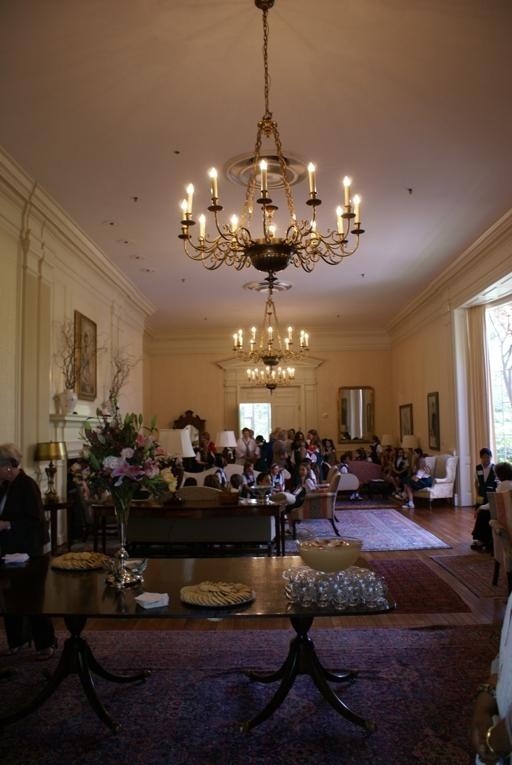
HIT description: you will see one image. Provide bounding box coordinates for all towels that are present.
[134,590,170,610]
[2,553,30,565]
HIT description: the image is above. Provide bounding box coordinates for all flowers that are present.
[192,432,218,455]
[68,392,179,526]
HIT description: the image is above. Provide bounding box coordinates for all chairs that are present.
[322,462,363,501]
[413,454,458,512]
[486,489,512,595]
[288,465,344,538]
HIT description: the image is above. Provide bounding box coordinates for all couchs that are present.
[108,464,296,559]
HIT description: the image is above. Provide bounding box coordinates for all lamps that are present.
[33,440,64,503]
[215,430,238,464]
[157,426,196,470]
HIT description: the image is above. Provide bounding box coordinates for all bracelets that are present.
[485,725,498,755]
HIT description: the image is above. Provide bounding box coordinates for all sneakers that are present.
[35,645,54,660]
[392,488,415,509]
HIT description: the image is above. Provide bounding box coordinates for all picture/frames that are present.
[74,310,98,401]
[427,391,440,451]
[399,403,413,441]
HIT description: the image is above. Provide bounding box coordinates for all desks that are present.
[40,498,67,556]
[36,556,397,735]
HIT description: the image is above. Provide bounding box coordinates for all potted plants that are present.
[52,318,108,412]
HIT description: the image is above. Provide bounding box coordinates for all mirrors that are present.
[337,385,376,444]
[173,410,206,462]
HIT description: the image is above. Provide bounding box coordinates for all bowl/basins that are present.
[295,532,364,576]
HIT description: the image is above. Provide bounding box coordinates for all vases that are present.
[103,509,144,589]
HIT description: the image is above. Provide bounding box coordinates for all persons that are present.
[197,427,436,539]
[470,460,512,550]
[475,447,498,511]
[0,442,58,661]
[471,583,512,765]
